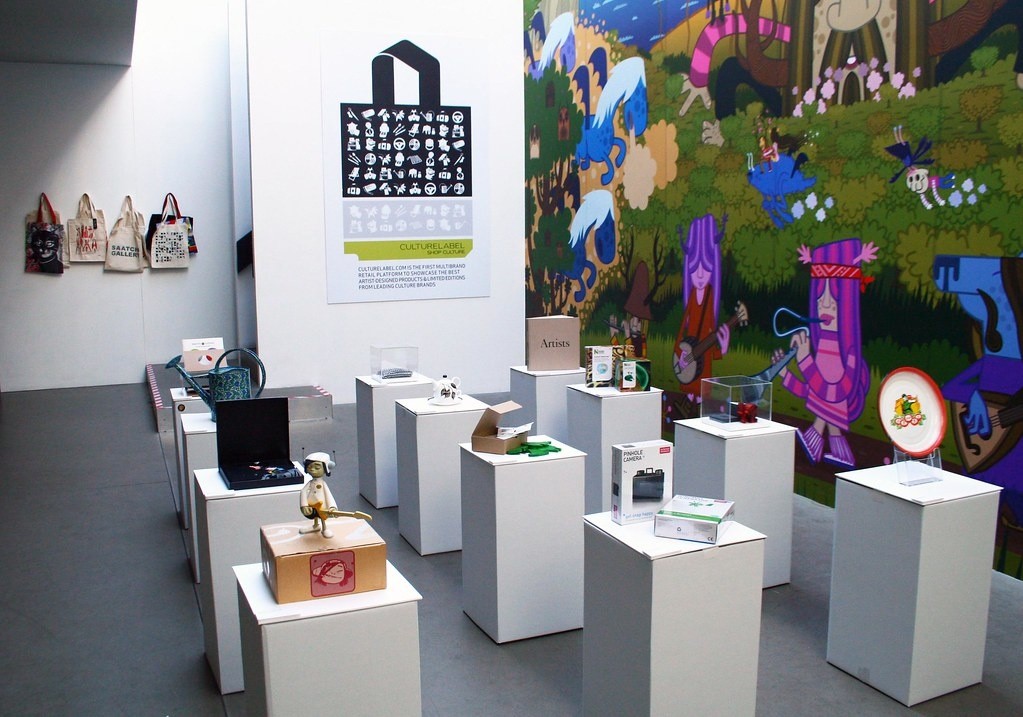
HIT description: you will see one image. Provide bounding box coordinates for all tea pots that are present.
[432,374,461,404]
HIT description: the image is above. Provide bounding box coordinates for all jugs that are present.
[164,348,266,422]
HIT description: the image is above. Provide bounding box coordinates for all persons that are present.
[299,452,338,538]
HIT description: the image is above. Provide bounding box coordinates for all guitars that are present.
[300,500,373,522]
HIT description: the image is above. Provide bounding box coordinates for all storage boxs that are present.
[654,494,735,544]
[611,439,674,524]
[261,515,388,604]
[614,359,650,393]
[367,345,418,382]
[215,399,304,491]
[469,401,527,455]
[700,376,774,430]
[585,344,635,387]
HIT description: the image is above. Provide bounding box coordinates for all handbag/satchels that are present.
[104,195,151,272]
[25,191,64,273]
[156,195,198,252]
[78,194,105,236]
[146,193,193,251]
[66,193,106,263]
[147,192,191,268]
[26,194,70,268]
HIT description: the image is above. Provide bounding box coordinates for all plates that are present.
[877,365,947,458]
[427,396,464,408]
